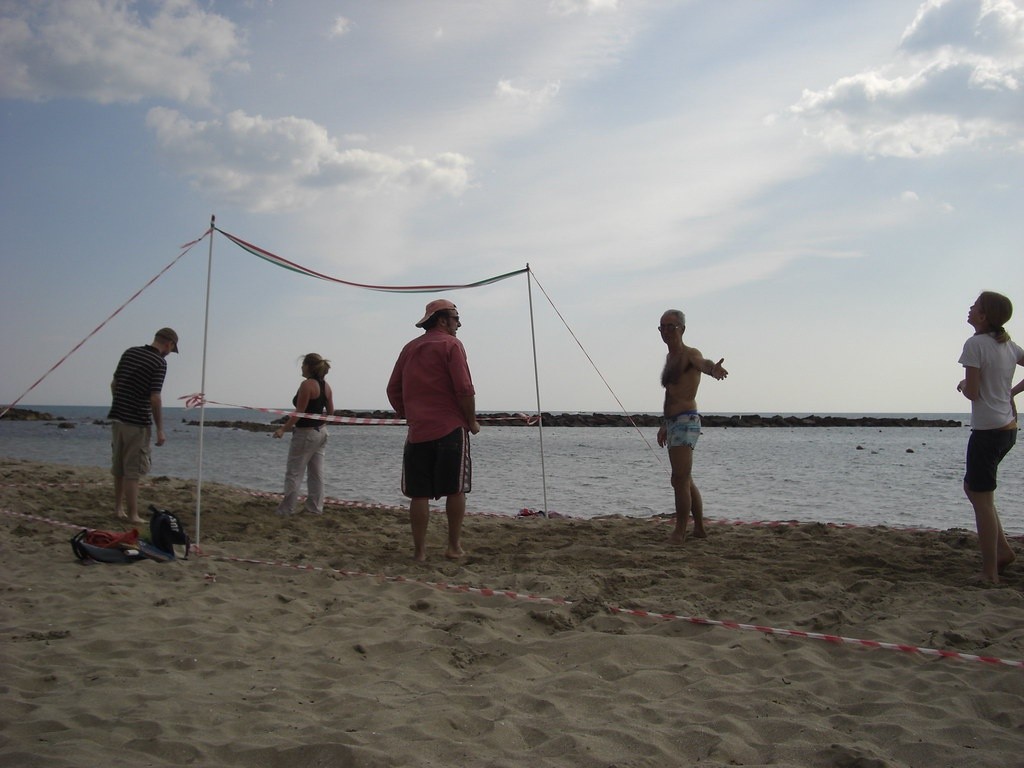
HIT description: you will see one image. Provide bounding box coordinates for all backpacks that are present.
[146,505,190,560]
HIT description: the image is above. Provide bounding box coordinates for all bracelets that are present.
[710,365,713,374]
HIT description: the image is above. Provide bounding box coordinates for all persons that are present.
[272,353,334,514]
[387,300,480,561]
[957,291,1024,584]
[108,328,179,522]
[657,310,728,544]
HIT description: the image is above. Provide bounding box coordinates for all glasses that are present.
[658,325,681,331]
[448,316,459,319]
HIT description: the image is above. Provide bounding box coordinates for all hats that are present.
[157,328,179,353]
[415,298,458,326]
